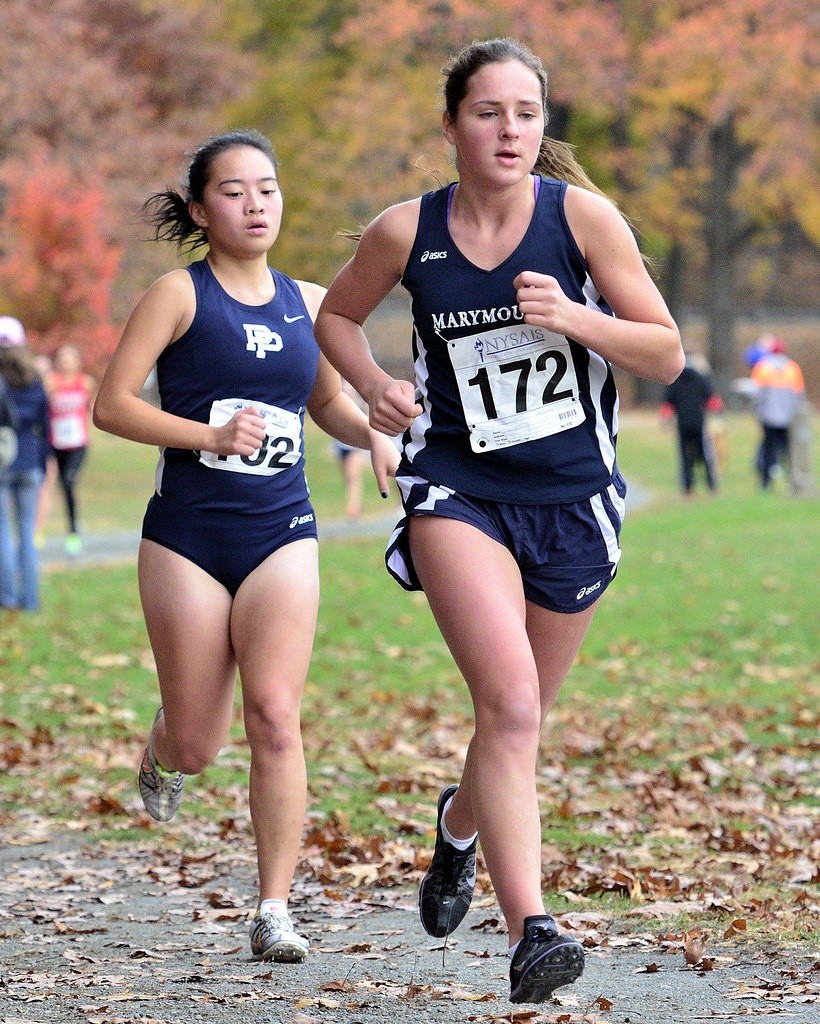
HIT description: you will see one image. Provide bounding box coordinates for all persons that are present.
[40,342,100,538]
[0,313,52,614]
[92,127,402,963]
[662,336,812,499]
[336,381,367,515]
[313,37,686,1003]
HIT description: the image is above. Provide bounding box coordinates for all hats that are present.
[0,316,27,347]
[743,347,763,368]
[759,333,784,351]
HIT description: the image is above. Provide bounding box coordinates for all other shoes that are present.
[33,533,47,549]
[66,533,82,555]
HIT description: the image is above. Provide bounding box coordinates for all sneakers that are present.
[507,915,585,1004]
[139,705,183,822]
[418,782,478,937]
[248,898,310,963]
